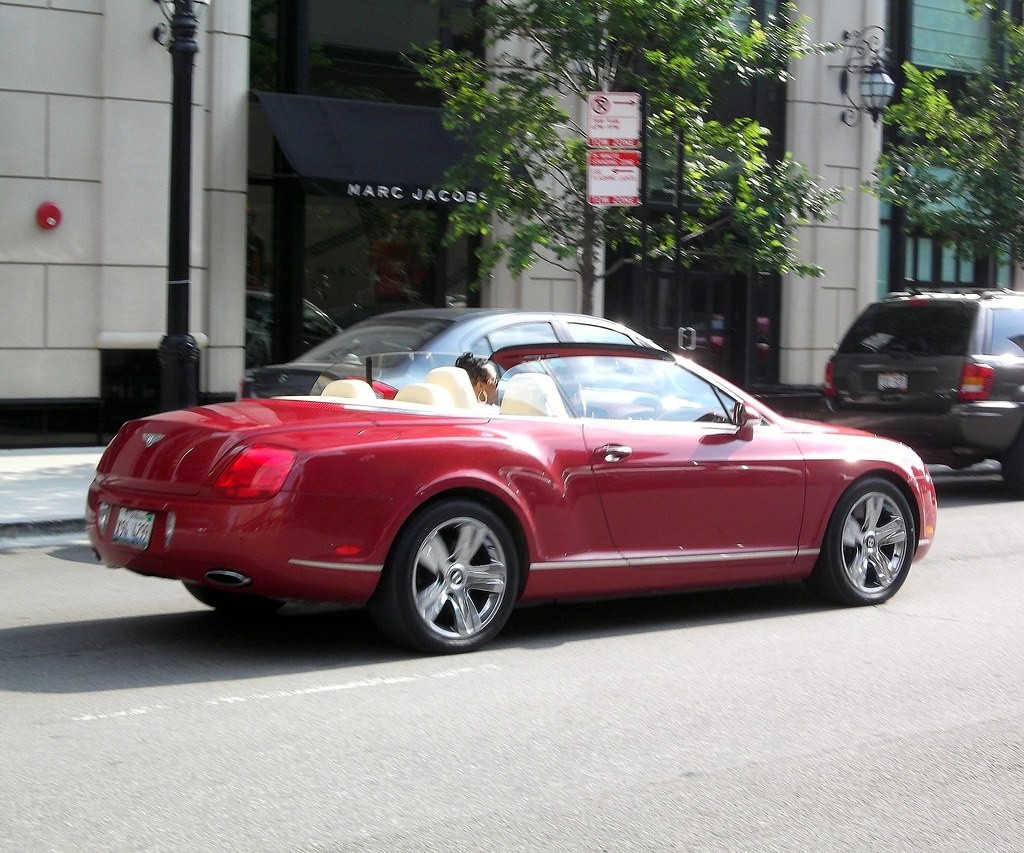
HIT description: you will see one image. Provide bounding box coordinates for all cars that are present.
[234,308,664,404]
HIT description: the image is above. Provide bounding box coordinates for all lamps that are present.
[826,24,895,130]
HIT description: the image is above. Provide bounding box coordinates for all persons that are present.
[456,351,501,410]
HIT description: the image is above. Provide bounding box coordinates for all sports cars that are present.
[85,339,936,655]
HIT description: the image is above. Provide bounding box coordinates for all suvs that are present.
[818,289,1024,499]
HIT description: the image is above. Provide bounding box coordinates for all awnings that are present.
[250,90,537,205]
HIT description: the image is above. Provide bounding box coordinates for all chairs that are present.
[321,368,570,418]
